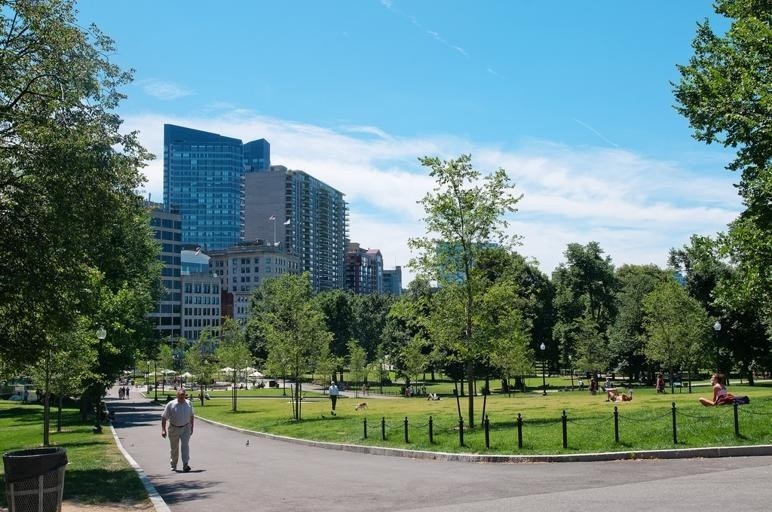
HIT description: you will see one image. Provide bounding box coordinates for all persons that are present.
[699,374,728,407]
[23,387,43,403]
[578,376,634,402]
[405,383,440,400]
[362,383,370,396]
[329,381,339,410]
[119,386,130,399]
[656,374,664,393]
[161,387,194,471]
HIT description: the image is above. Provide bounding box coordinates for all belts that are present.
[170,423,189,428]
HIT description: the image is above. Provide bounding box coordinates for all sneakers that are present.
[183,465,191,471]
[172,468,176,471]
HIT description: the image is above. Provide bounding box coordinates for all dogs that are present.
[355,402,368,411]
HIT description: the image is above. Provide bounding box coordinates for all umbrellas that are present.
[149,366,265,380]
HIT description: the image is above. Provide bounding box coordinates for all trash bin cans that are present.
[3,447,66,511]
